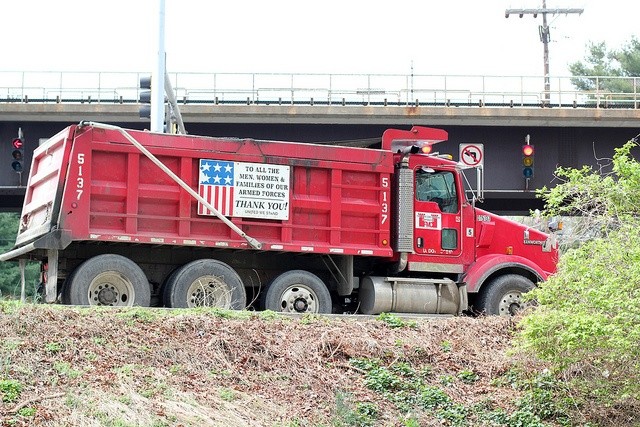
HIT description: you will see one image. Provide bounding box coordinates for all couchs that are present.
[138,74,152,117]
[520,143,536,180]
[11,138,26,175]
